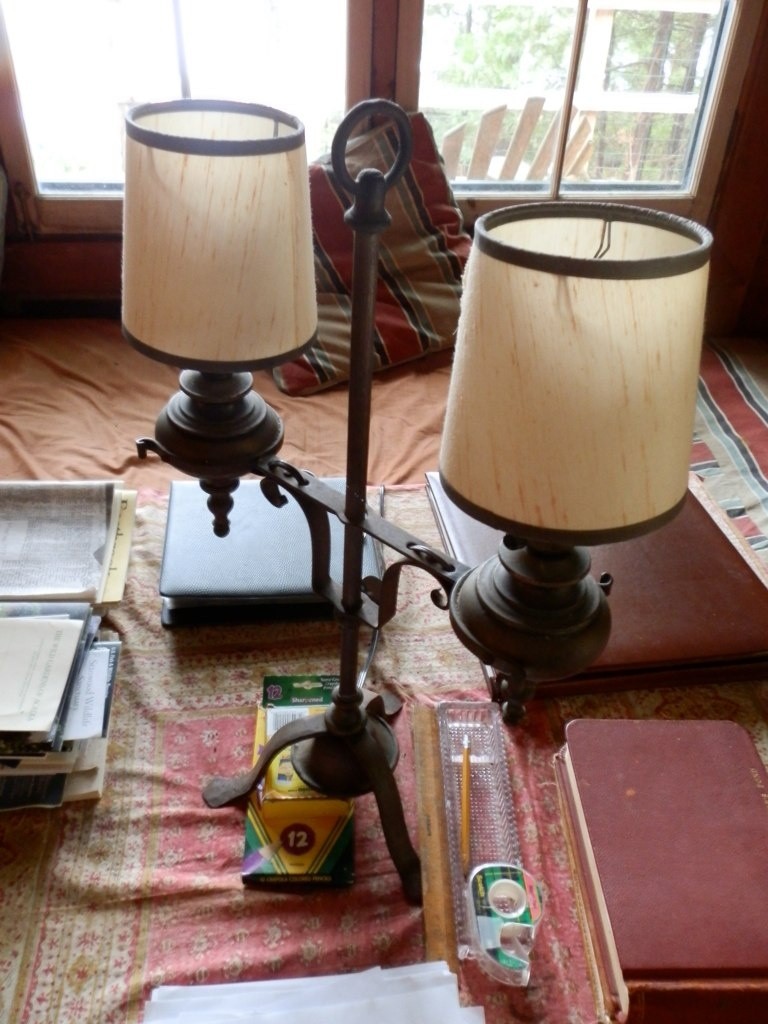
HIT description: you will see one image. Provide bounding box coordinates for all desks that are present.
[0,475,768,1024]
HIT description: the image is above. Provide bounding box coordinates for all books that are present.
[1,480,140,812]
[553,717,768,1024]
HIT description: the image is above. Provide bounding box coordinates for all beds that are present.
[0,296,768,576]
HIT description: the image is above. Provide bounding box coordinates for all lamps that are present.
[77,97,712,905]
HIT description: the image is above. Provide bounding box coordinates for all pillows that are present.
[270,115,470,396]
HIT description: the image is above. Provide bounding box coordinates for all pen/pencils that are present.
[460,732,471,880]
